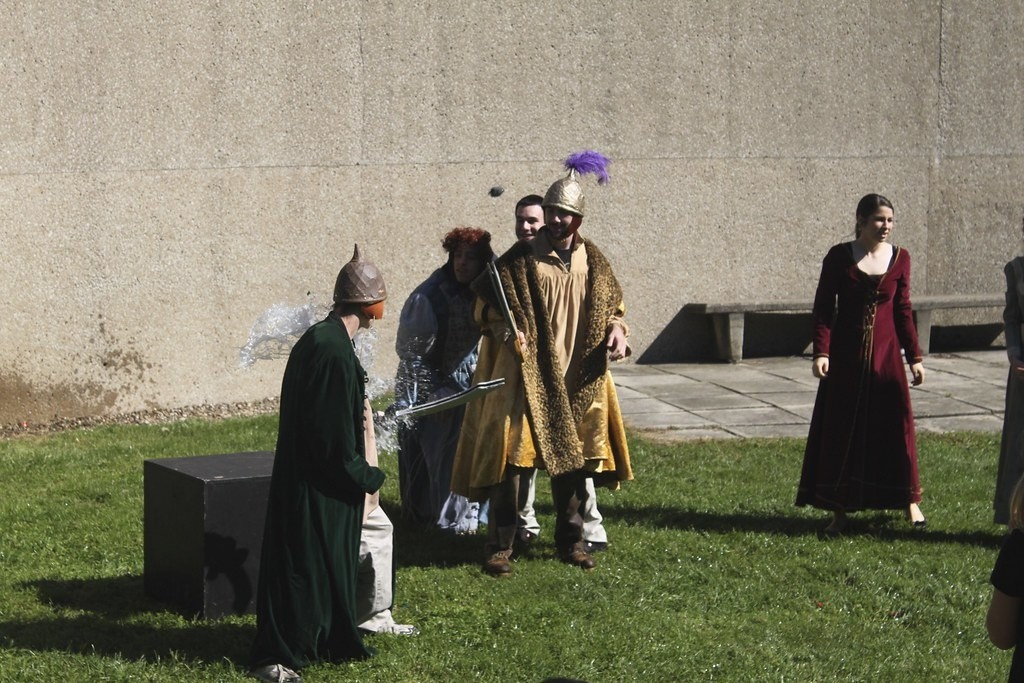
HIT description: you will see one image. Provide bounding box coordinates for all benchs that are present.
[685,293,1005,364]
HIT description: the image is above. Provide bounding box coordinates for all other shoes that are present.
[583,542,609,552]
[906,503,926,527]
[520,529,533,542]
[359,624,420,638]
[824,514,847,533]
[253,663,301,683]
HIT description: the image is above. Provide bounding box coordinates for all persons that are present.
[387,227,501,533]
[992,255,1023,523]
[250,241,420,683]
[447,178,632,577]
[794,193,926,527]
[987,476,1024,683]
[515,194,545,240]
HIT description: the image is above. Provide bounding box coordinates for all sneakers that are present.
[486,549,511,576]
[567,552,594,567]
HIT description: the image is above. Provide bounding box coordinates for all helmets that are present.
[333,243,387,320]
[541,150,613,218]
